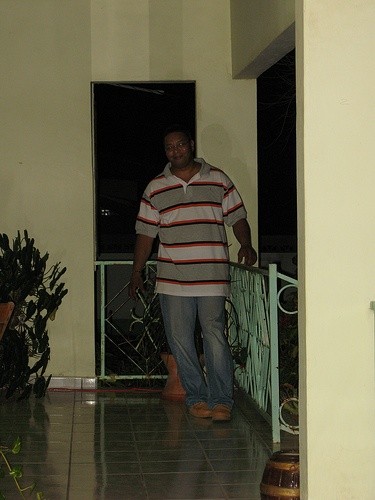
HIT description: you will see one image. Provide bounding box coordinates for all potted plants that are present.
[138,264,248,401]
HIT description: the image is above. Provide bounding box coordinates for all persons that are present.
[129,126,257,422]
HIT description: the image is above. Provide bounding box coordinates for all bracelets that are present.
[133,269,143,273]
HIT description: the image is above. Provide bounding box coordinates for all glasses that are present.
[165,141,190,151]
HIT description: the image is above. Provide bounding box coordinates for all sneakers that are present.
[190,402,212,418]
[212,404,232,422]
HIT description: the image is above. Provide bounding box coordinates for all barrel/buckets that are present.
[260,449,300,500]
